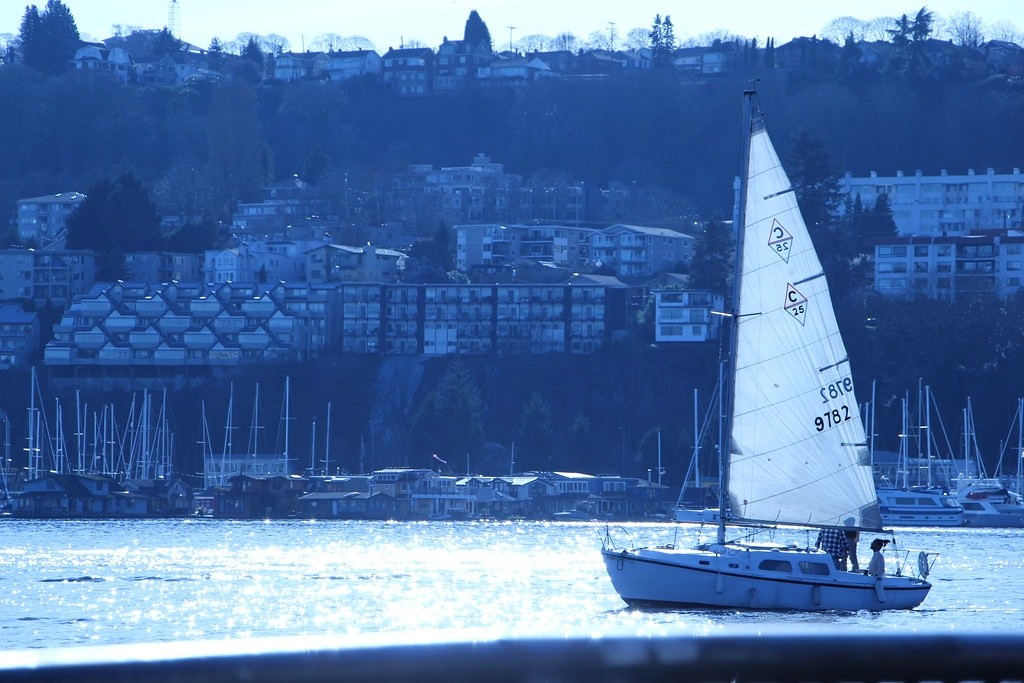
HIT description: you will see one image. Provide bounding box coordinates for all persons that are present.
[867,539,891,576]
[841,517,860,572]
[815,528,849,570]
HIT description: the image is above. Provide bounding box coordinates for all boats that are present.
[551,501,598,524]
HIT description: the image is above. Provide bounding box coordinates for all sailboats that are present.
[600,79,940,612]
[669,361,1023,526]
